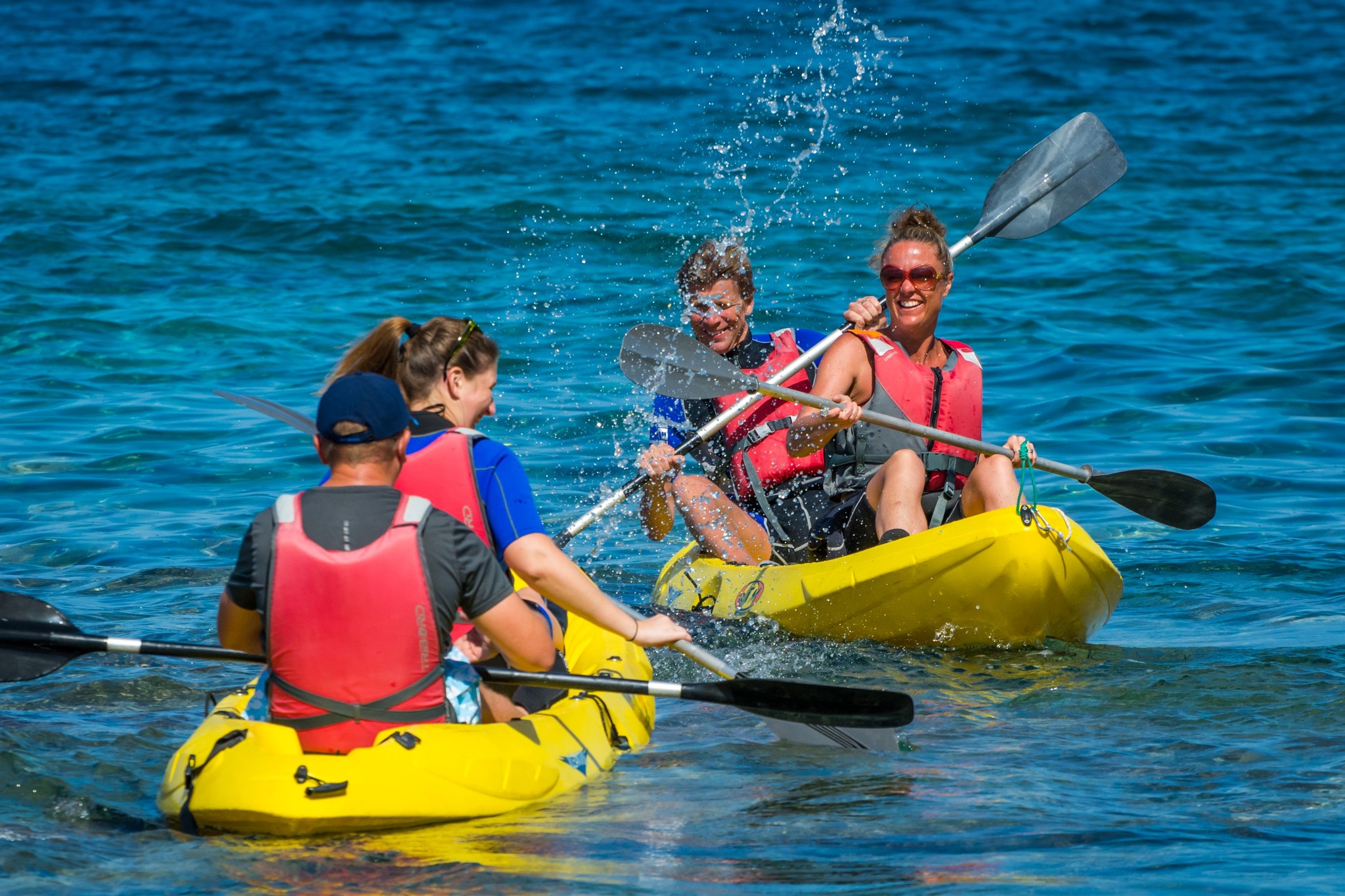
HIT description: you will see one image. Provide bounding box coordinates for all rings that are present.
[862,300,867,304]
[1027,448,1033,454]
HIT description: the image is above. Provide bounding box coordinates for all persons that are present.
[218,314,692,754]
[785,202,1037,562]
[638,233,889,566]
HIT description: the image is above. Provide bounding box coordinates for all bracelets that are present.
[627,620,638,642]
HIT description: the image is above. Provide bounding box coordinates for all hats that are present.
[316,371,422,445]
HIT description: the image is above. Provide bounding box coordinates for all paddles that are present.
[211,385,866,749]
[617,322,1217,530]
[550,110,1129,549]
[0,589,914,729]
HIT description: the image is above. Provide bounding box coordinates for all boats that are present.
[154,562,659,835]
[650,501,1126,653]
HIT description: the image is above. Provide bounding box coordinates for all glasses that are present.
[880,263,952,290]
[444,317,483,382]
